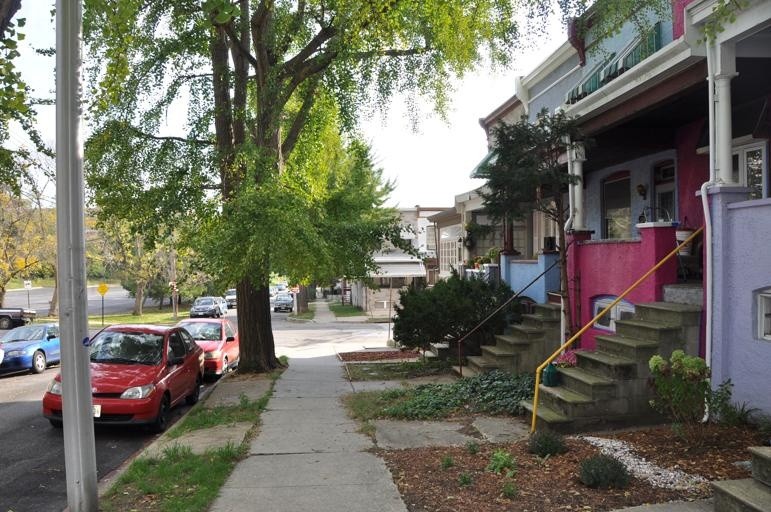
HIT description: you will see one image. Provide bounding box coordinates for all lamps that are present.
[636,183,647,200]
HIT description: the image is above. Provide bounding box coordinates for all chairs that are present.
[676,228,700,283]
[119,336,160,357]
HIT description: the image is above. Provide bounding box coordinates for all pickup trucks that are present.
[0,306,37,330]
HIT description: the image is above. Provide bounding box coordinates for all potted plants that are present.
[458,231,502,270]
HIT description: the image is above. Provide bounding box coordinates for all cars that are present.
[188,279,298,319]
[0,322,61,376]
[174,318,241,379]
[41,324,206,433]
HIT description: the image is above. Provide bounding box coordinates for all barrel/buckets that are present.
[675,231,693,256]
